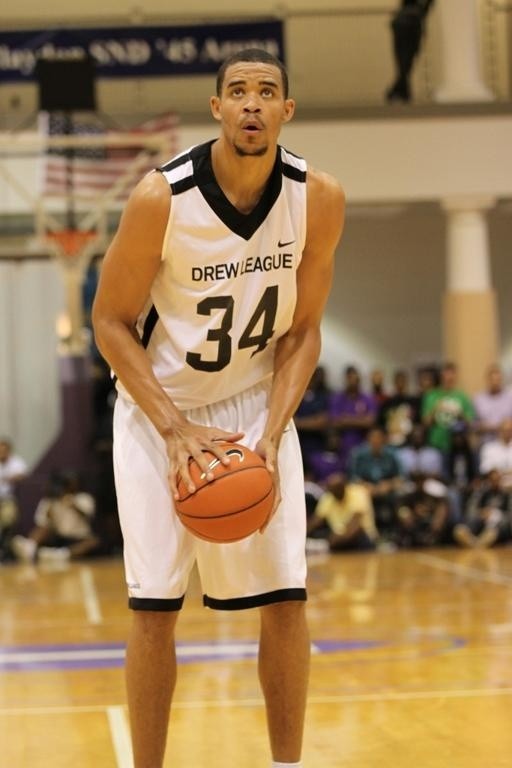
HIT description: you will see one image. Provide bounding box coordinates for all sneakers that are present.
[452,524,498,548]
[306,538,332,554]
[13,537,70,563]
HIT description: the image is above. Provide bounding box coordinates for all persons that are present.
[0,364,512,566]
[92,50,348,767]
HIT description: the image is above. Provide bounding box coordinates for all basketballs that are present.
[172,441,275,543]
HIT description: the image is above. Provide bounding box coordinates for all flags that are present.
[36,111,177,204]
